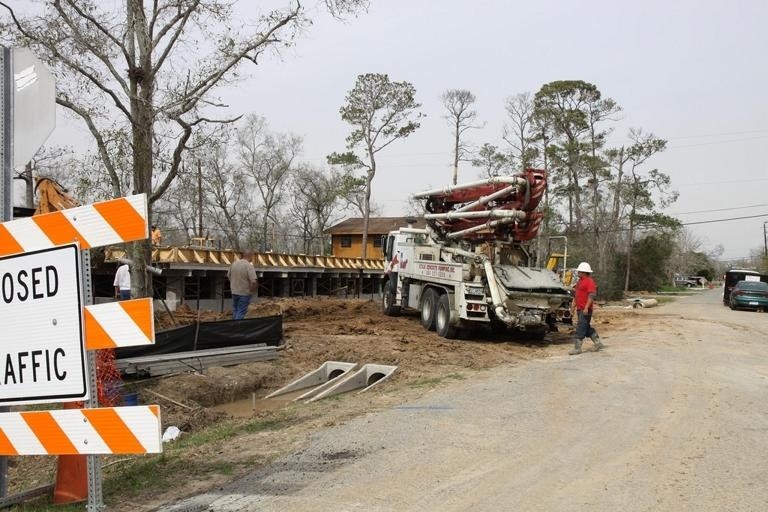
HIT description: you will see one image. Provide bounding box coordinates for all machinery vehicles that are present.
[377,165,575,341]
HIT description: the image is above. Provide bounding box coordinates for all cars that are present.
[726,280,767,314]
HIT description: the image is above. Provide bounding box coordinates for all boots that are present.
[590,333,603,352]
[567,339,583,355]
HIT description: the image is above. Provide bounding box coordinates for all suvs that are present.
[674,276,696,288]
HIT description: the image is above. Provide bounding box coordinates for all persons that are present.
[568,262,603,355]
[151,225,161,247]
[224,249,257,320]
[113,263,131,301]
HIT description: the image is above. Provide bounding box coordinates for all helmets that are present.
[573,262,593,274]
